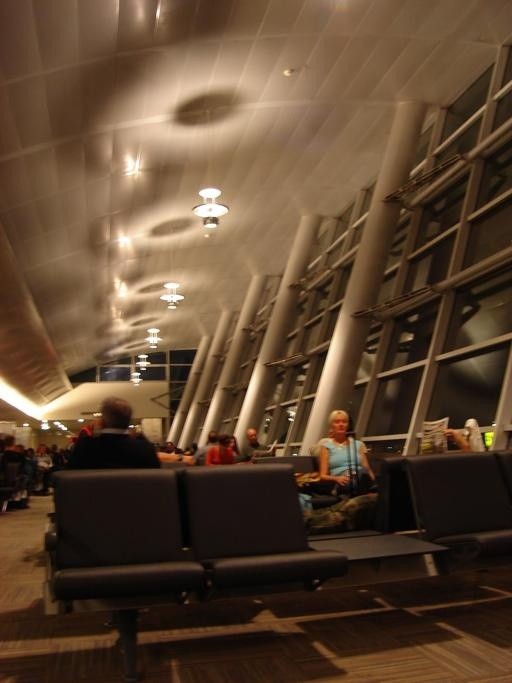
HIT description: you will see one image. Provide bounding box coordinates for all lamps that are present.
[130,185,231,388]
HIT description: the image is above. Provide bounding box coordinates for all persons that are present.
[65,396,161,470]
[443,427,471,453]
[0,433,76,510]
[156,428,275,466]
[318,410,377,497]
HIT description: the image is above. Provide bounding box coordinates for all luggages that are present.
[336,432,390,534]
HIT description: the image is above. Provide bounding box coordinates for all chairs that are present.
[42,449,512,683]
